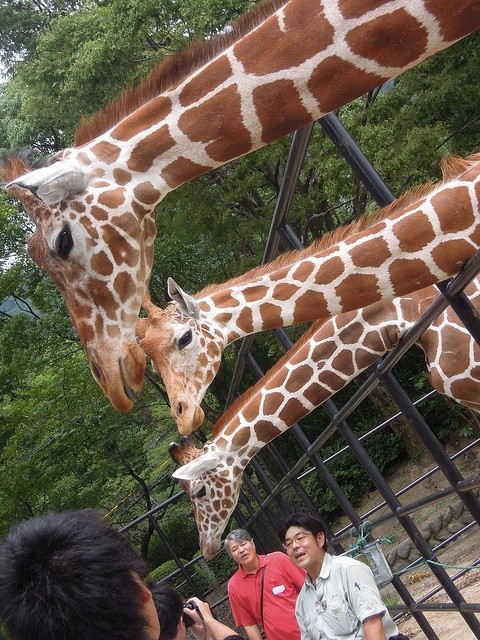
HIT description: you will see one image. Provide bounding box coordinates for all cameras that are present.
[182,603,203,628]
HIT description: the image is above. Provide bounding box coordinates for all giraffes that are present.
[132,149,479,436]
[0,2,479,416]
[168,266,478,564]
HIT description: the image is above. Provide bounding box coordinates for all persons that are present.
[149,584,243,639]
[1,508,161,640]
[278,513,408,639]
[224,529,308,640]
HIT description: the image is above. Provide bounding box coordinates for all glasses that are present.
[282,532,312,550]
